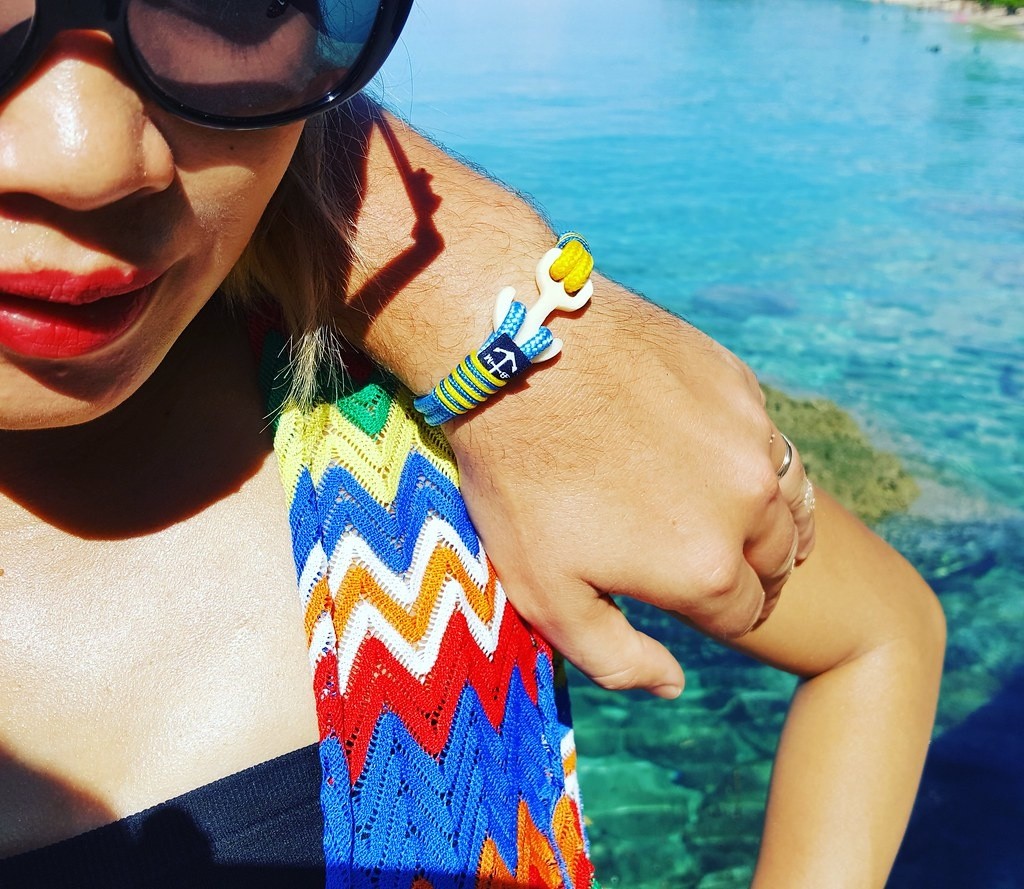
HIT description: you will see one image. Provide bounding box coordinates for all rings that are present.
[775,431,792,480]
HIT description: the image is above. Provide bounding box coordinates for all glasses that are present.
[0,0,415,133]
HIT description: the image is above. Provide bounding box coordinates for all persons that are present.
[0,0,950,889]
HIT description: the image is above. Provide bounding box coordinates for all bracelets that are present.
[407,233,598,435]
